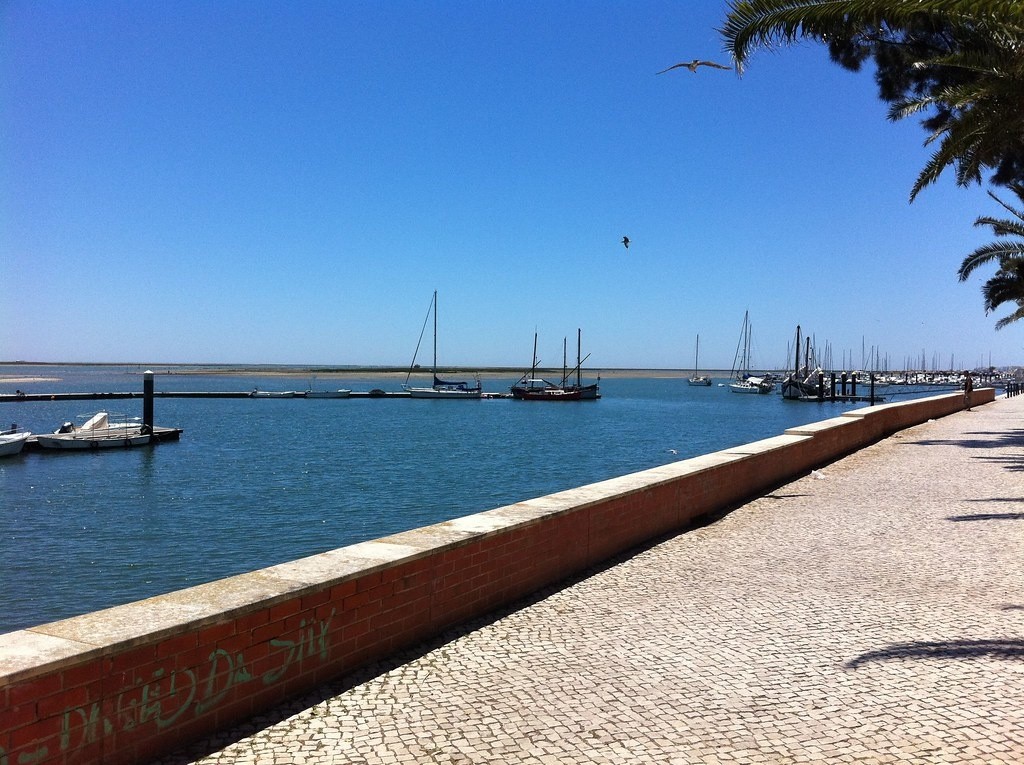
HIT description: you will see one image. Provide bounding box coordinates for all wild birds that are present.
[620,236,632,250]
[656,60,733,74]
[669,450,681,455]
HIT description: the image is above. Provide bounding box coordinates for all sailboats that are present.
[875,351,897,381]
[400,290,483,398]
[736,324,777,392]
[892,356,909,385]
[862,346,890,387]
[687,334,711,386]
[727,310,761,394]
[909,348,996,386]
[781,325,832,399]
[852,370,868,377]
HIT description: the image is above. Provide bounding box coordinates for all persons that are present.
[964,370,973,411]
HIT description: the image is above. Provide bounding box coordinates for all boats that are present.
[507,328,599,400]
[773,374,784,382]
[36,434,152,448]
[253,390,295,398]
[717,383,726,387]
[0,431,31,458]
[305,389,351,398]
